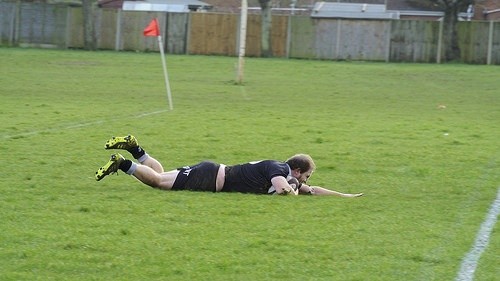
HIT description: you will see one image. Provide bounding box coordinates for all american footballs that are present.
[268,176,299,195]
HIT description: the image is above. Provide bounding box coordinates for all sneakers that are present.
[94,152,125,182]
[104,134,139,150]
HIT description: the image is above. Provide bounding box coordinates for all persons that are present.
[94,134,364,199]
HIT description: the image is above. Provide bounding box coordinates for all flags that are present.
[143,18,159,37]
